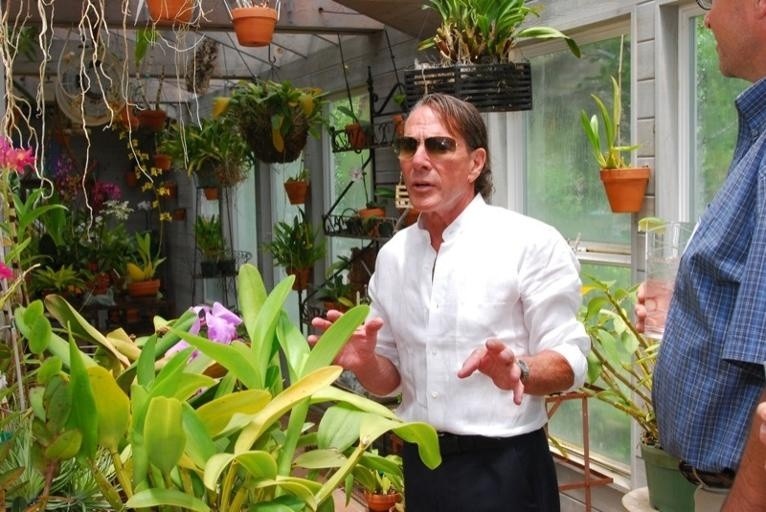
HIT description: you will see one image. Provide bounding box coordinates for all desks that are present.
[617,486,657,512]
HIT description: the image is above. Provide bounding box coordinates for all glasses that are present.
[393,136,464,159]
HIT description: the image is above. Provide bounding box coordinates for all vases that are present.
[230,8,280,47]
[142,0,197,27]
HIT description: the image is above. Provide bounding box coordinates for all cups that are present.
[640,218,694,340]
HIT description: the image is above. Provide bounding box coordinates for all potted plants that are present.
[579,35,651,215]
[209,81,328,167]
[122,230,167,306]
[283,167,312,206]
[356,183,391,217]
[357,455,404,511]
[191,213,242,278]
[398,0,582,112]
[337,103,372,149]
[560,245,706,512]
[265,215,333,294]
[139,99,189,224]
[158,119,252,200]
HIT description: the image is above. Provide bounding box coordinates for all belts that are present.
[680,462,735,488]
[438,434,500,445]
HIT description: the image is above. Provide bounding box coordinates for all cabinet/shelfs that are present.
[294,58,534,340]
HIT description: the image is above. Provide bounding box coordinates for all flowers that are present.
[0,134,35,182]
[42,160,135,225]
[106,104,176,224]
[169,299,247,359]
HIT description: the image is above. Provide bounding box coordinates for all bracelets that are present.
[514,358,530,384]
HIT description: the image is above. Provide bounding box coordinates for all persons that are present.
[648,0,766,512]
[305,91,596,512]
